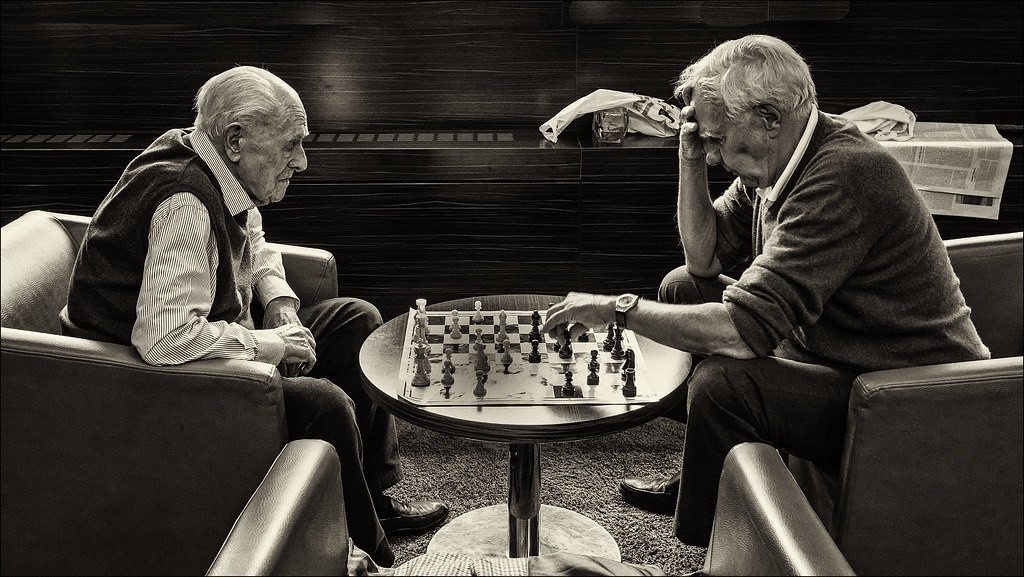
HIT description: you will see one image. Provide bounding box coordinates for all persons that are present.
[539,35,991,549]
[59,66,446,569]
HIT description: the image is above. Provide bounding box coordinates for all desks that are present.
[357,295,694,562]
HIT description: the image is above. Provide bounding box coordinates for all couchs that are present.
[0,212,339,577]
[203,438,857,577]
[787,231,1024,577]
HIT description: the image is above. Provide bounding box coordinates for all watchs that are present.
[616,293,639,328]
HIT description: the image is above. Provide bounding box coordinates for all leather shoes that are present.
[379,495,450,536]
[618,476,670,510]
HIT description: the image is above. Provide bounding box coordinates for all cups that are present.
[594,107,629,144]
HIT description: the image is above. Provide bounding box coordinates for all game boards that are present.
[395,307,661,407]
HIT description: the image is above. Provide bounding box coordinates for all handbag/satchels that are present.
[539,89,681,143]
